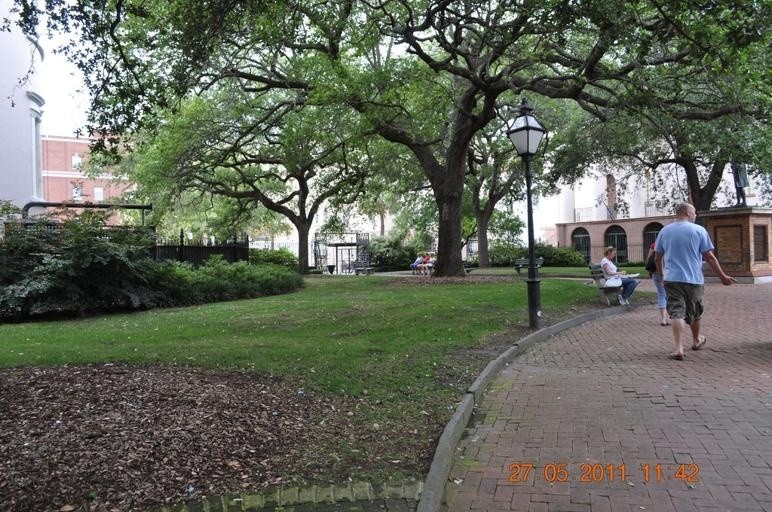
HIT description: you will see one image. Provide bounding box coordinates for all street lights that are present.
[505,96,548,334]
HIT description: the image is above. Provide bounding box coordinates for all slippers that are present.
[667,351,684,361]
[691,335,707,350]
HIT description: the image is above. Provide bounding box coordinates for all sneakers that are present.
[617,293,630,306]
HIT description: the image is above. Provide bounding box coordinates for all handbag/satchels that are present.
[645,248,658,273]
[602,276,623,288]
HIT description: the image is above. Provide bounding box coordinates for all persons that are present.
[409,253,422,274]
[423,254,436,275]
[729,143,750,206]
[418,253,430,274]
[652,202,739,356]
[647,240,668,326]
[600,245,640,305]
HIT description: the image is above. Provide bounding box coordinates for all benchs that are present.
[350,260,374,275]
[588,264,625,306]
[514,259,544,274]
